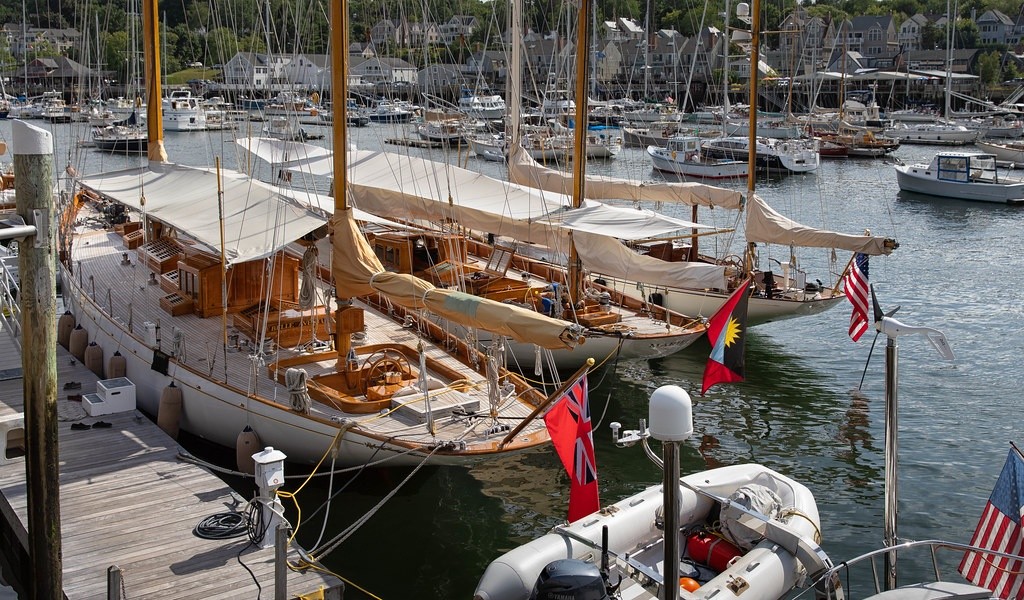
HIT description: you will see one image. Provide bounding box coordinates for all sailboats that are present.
[234,2,713,372]
[0,1,1024,178]
[56,0,574,474]
[505,1,899,331]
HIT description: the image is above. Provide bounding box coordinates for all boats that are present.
[790,537,1024,600]
[974,138,1024,165]
[469,461,823,600]
[891,154,1024,208]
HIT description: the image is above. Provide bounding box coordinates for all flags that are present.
[698,273,750,400]
[956,446,1024,600]
[841,247,872,342]
[528,368,603,525]
